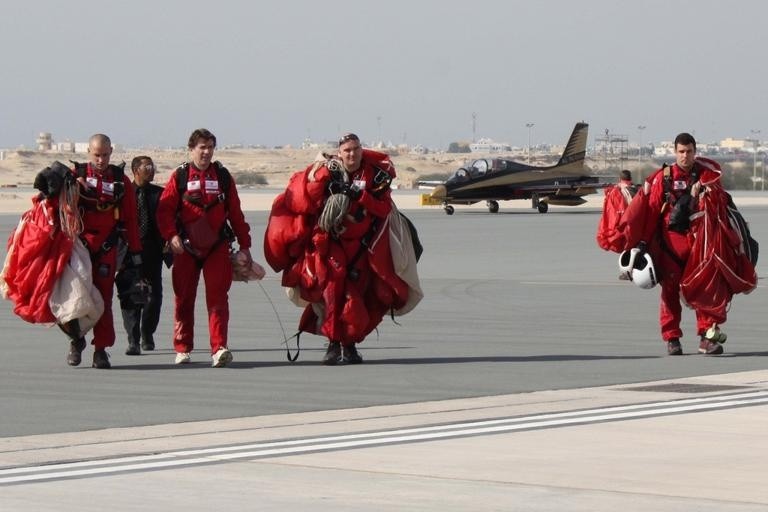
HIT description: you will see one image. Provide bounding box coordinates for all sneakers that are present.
[320,343,343,364]
[342,345,362,363]
[175,351,191,364]
[211,346,234,368]
[667,338,683,356]
[141,332,155,351]
[66,335,87,366]
[698,337,724,354]
[91,351,112,369]
[124,342,141,355]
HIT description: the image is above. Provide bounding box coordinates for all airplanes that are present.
[428,121,618,214]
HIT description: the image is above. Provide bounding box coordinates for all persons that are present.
[597,169,642,280]
[115,156,165,355]
[312,133,393,365]
[155,129,251,368]
[634,133,723,355]
[57,133,143,369]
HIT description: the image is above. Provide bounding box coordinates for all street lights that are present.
[637,125,645,183]
[749,128,760,191]
[525,122,534,166]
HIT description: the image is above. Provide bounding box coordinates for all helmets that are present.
[632,251,659,290]
[618,248,640,281]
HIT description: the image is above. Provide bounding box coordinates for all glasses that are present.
[139,164,157,171]
